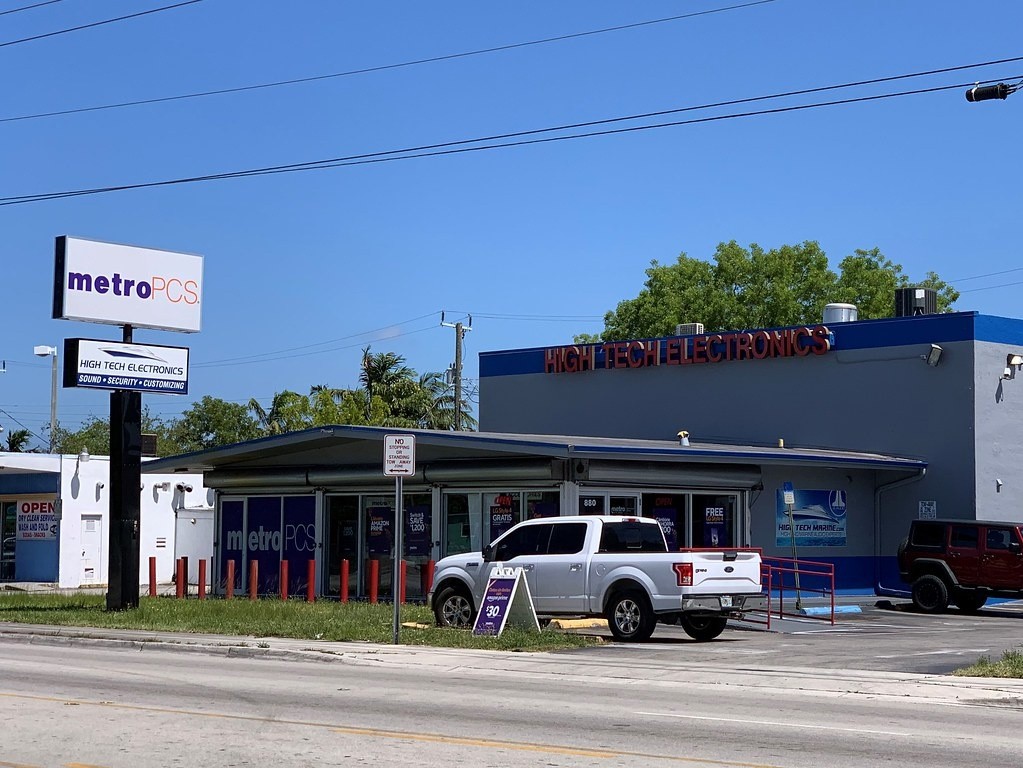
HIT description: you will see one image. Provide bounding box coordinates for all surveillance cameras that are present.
[98,483,104,488]
[176,482,194,492]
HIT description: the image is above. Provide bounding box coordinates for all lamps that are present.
[76,450,89,475]
[1007,353,1023,365]
[176,482,193,494]
[925,344,943,368]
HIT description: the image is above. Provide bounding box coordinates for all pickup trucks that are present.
[428,515,768,643]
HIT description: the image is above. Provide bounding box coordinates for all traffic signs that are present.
[383,434,416,478]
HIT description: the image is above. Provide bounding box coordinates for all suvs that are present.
[897,519,1023,615]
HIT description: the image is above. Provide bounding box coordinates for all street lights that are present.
[33,345,58,453]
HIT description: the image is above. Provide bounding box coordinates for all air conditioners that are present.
[676,323,704,336]
